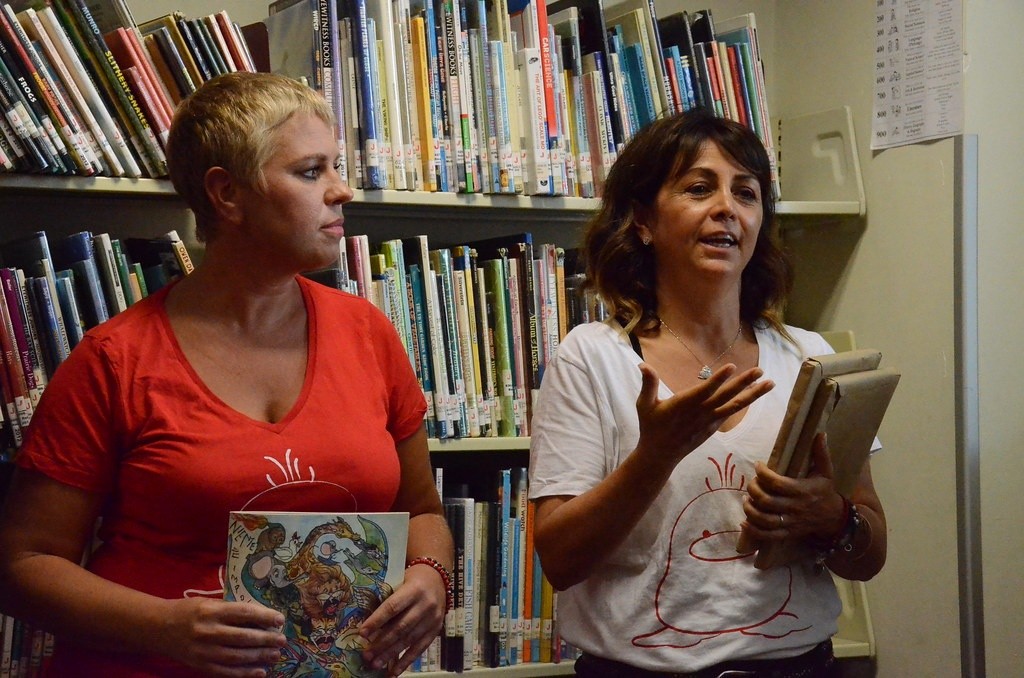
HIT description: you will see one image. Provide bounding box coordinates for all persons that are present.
[527,112,888,678]
[0,72,454,678]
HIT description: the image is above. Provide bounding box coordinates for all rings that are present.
[779,514,784,527]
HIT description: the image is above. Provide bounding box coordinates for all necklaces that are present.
[660,319,742,379]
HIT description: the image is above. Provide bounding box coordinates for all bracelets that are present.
[408,558,453,614]
[815,500,873,576]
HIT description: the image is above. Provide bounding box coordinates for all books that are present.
[0,516,103,678]
[400,464,582,673]
[307,234,615,440]
[0,0,782,204]
[0,229,195,450]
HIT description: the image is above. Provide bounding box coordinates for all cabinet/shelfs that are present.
[0,0,877,678]
